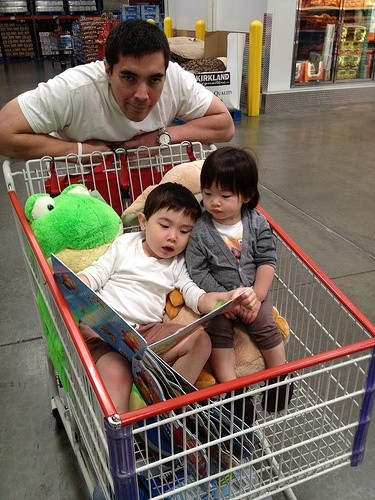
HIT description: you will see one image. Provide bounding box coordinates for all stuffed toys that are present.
[24,159,290,412]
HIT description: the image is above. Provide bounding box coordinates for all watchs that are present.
[157,127,172,151]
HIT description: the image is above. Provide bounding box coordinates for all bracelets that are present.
[76,142,84,164]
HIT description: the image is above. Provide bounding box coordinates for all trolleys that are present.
[1,142,375,500]
[48,32,78,69]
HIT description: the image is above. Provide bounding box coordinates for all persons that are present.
[43,183,257,499]
[0,19,236,165]
[183,147,295,456]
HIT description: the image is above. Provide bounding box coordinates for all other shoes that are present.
[132,416,172,458]
[223,385,256,429]
[259,371,294,413]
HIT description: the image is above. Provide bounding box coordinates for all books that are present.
[47,249,259,493]
